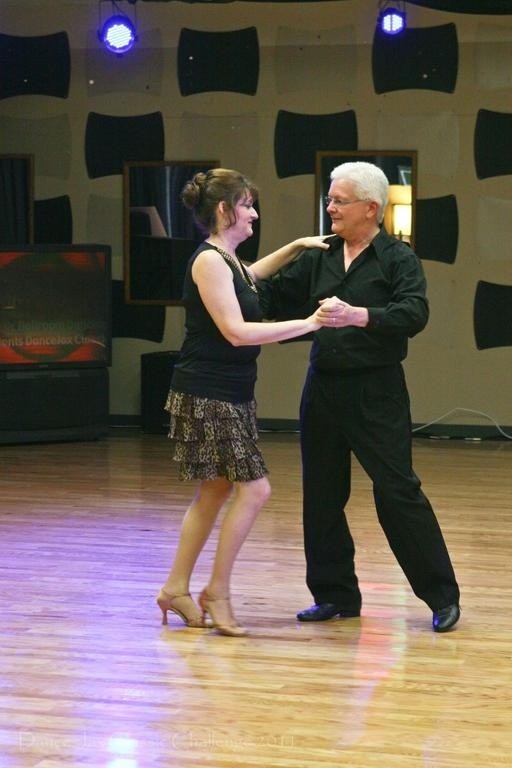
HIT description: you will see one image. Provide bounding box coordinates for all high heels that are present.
[198,585,249,638]
[155,586,213,631]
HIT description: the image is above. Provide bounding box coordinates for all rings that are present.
[332,318,335,323]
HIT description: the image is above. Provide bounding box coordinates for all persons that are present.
[157,169,337,638]
[240,159,460,632]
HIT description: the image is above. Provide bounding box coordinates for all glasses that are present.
[323,193,373,206]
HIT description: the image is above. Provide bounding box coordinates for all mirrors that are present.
[121,158,222,306]
[314,148,418,256]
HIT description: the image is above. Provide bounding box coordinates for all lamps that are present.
[377,2,407,36]
[97,0,140,55]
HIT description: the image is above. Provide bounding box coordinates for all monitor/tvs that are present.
[0,244,113,371]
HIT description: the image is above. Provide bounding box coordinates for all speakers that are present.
[141,349,183,434]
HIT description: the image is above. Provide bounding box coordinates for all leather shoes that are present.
[431,601,461,633]
[294,602,362,622]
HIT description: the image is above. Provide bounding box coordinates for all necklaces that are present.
[216,248,258,294]
